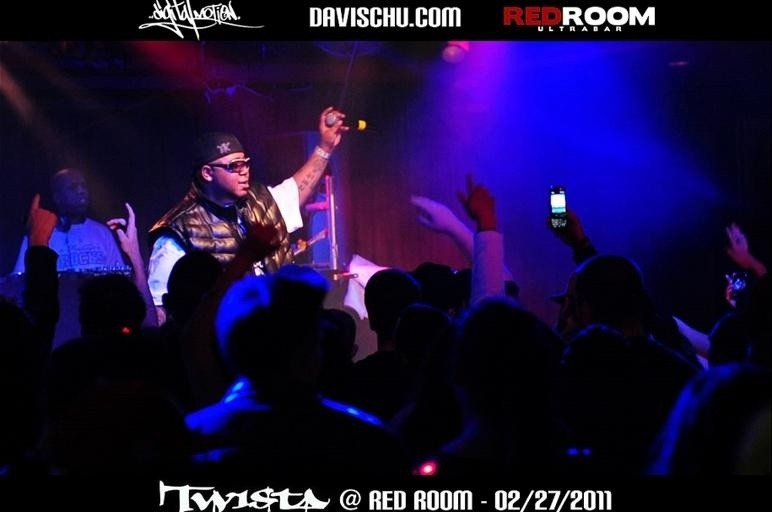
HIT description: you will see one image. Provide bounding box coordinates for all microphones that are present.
[322,112,367,131]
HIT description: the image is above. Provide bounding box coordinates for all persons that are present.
[1,104,771,508]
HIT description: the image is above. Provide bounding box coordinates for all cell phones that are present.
[549,186,568,229]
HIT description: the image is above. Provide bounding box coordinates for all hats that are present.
[194,132,244,167]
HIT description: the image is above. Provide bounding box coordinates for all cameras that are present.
[724,269,751,299]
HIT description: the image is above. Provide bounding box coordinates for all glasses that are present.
[207,157,251,173]
